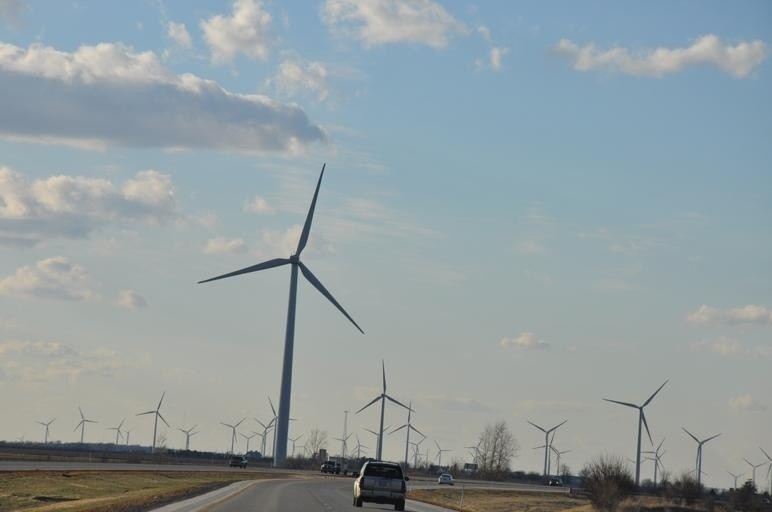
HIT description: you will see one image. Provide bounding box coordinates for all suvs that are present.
[353,461,407,511]
[320,461,348,475]
[228,456,248,469]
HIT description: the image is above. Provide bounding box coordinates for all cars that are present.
[549,480,560,486]
[439,474,455,485]
